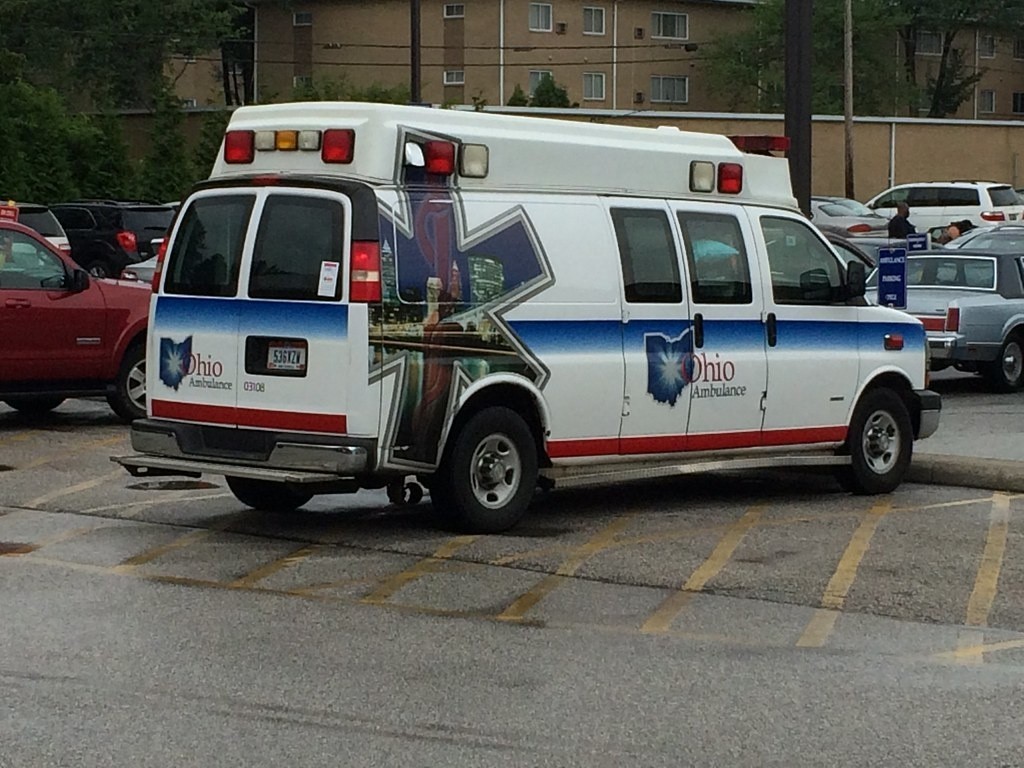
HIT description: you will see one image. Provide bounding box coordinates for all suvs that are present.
[863,179,1024,236]
[0,219,155,421]
[1,199,185,287]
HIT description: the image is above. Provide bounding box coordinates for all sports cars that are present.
[862,247,1024,394]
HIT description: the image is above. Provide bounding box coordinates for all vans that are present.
[110,96,942,531]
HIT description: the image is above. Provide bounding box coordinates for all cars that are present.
[803,197,1024,283]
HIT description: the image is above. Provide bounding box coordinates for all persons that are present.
[941,223,970,247]
[887,200,918,240]
